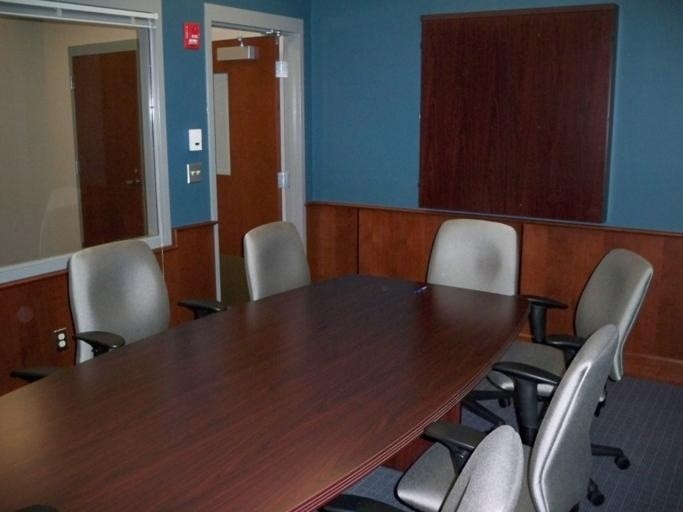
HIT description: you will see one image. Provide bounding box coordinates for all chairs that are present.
[244,220,311,302]
[426,215,522,296]
[476,244,655,505]
[65,238,226,364]
[393,324,619,512]
[438,425,524,511]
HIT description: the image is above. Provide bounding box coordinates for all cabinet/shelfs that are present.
[419,3,620,223]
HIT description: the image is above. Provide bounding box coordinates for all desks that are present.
[0,273,534,512]
